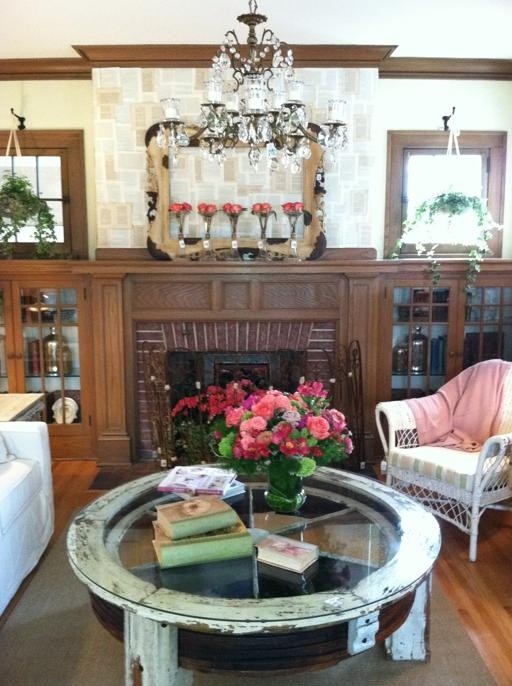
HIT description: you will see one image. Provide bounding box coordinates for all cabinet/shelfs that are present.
[0,259,98,461]
[375,258,512,464]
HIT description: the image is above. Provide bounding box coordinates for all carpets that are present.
[0,502,493,685]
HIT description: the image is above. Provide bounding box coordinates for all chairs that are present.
[373,358,512,562]
[0,421,56,617]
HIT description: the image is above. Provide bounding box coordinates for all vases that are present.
[264,458,308,512]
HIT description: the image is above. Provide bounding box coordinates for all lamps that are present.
[156,0,350,175]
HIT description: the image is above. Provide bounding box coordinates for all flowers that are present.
[209,377,355,478]
[170,379,258,463]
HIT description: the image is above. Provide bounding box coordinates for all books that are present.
[252,534,319,574]
[150,464,254,574]
